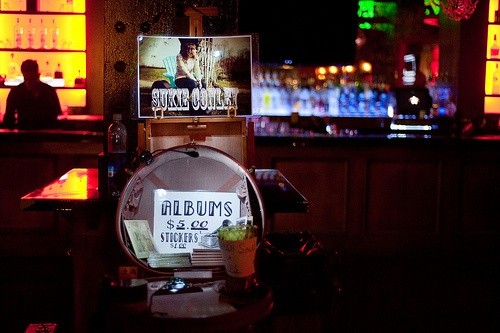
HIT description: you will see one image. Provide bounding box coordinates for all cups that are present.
[217,226,258,277]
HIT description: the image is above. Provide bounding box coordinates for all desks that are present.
[18,167,309,333]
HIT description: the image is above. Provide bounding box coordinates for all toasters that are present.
[107,114,127,153]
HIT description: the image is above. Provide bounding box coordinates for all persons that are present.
[2,59,63,130]
[174,39,208,114]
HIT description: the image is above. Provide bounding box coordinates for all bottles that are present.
[26,18,36,48]
[13,18,23,48]
[76,70,81,78]
[254,69,450,117]
[54,64,63,79]
[44,61,52,77]
[51,19,59,49]
[39,19,48,48]
[8,53,17,79]
